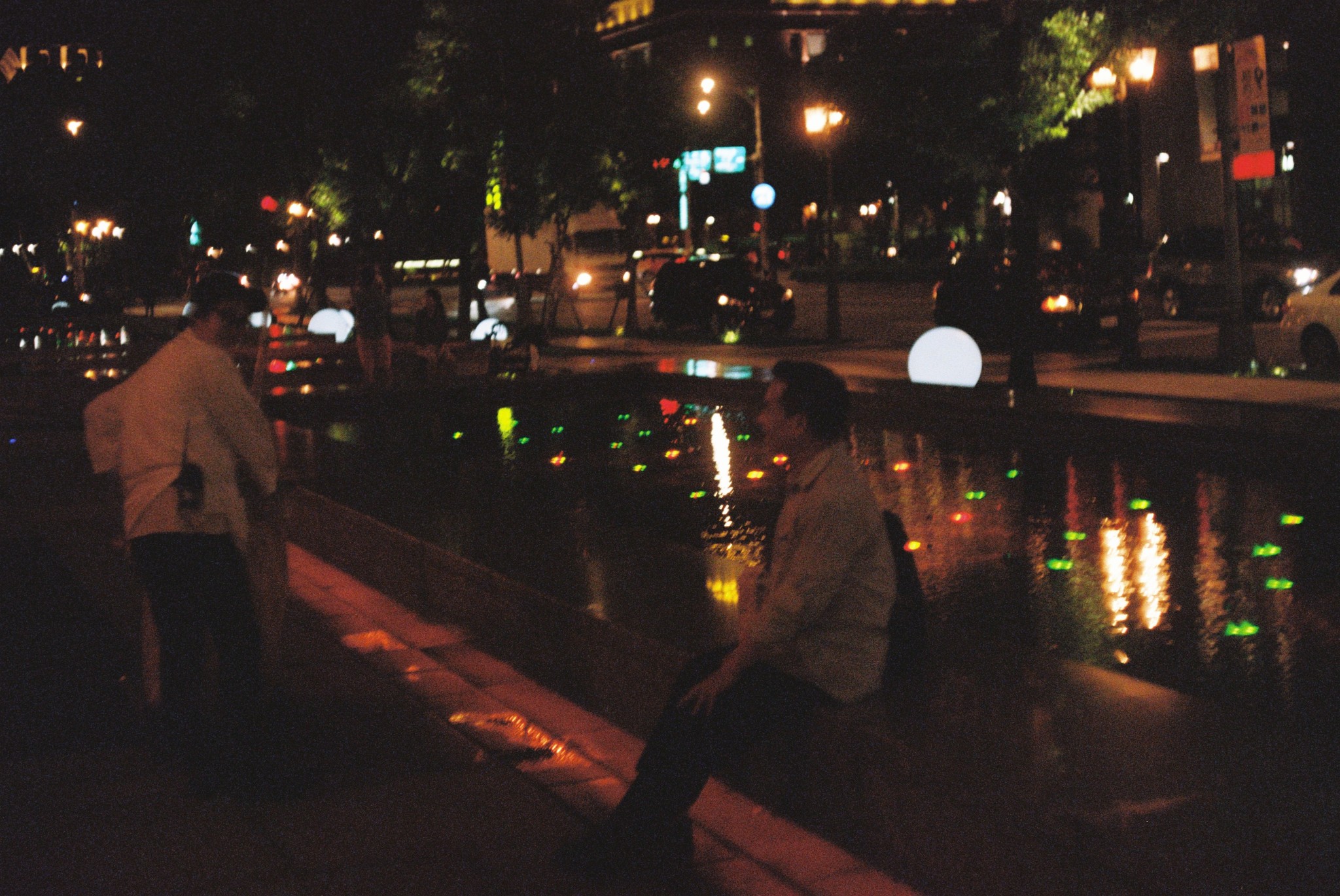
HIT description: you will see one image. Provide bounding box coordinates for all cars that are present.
[929,249,1144,356]
[1275,271,1340,376]
[562,227,797,333]
[454,269,555,335]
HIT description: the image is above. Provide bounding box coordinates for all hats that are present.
[189,272,268,312]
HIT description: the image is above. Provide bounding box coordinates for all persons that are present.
[410,289,452,392]
[540,358,897,896]
[356,261,397,395]
[81,273,321,798]
[250,274,337,489]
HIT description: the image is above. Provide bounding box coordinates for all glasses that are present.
[206,305,242,325]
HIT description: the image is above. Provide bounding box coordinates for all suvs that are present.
[1145,224,1340,324]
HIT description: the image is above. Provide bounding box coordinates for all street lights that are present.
[693,72,769,271]
[803,102,852,342]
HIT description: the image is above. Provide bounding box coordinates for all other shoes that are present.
[560,814,694,874]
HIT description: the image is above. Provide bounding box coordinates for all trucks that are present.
[481,195,635,304]
